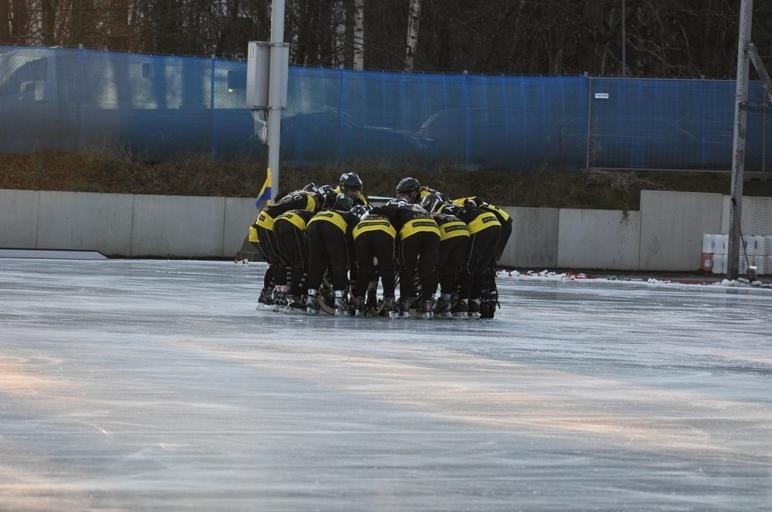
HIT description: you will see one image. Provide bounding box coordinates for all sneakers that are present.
[452,299,496,312]
[259,288,321,308]
[335,290,378,310]
[384,296,451,311]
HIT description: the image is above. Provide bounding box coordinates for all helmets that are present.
[387,198,408,209]
[340,173,362,189]
[351,203,375,218]
[397,177,420,201]
[304,182,352,211]
[420,191,450,211]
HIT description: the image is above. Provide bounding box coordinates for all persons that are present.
[248,171,513,317]
